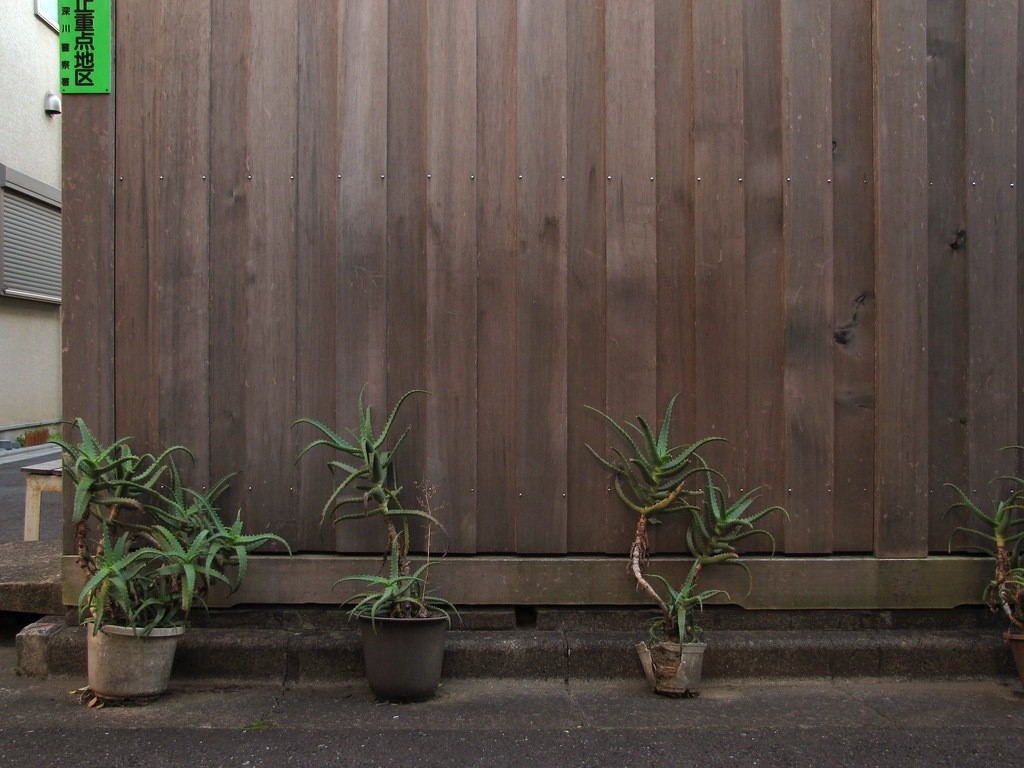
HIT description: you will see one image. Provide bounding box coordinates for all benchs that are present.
[20,460,62,541]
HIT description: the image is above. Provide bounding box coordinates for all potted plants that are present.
[49,420,290,703]
[573,395,791,693]
[292,388,451,706]
[935,444,1024,678]
